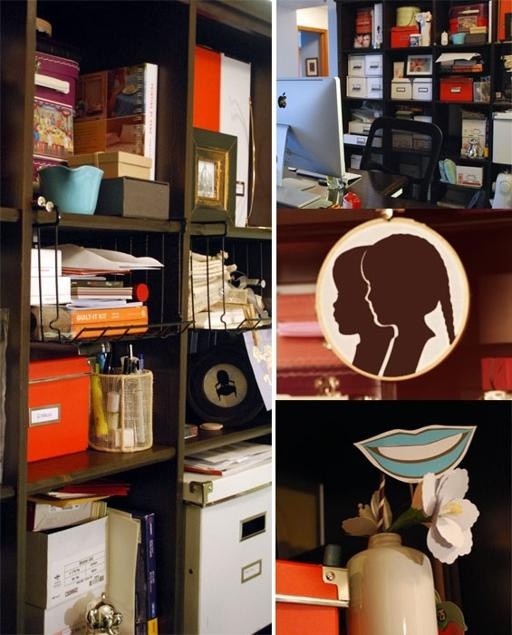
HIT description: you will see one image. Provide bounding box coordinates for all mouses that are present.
[287,167,296,171]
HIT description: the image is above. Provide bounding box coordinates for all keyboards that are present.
[296,169,363,185]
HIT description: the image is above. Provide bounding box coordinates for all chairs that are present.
[361,117,444,203]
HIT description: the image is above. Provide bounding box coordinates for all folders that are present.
[342,172,362,189]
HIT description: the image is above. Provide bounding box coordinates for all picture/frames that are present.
[404,54,433,77]
[306,58,318,77]
[193,128,237,227]
[190,343,266,426]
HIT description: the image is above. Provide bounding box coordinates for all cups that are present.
[36,165,104,216]
[450,32,466,45]
[409,34,422,47]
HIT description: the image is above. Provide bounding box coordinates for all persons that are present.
[361,34,370,48]
[374,25,382,47]
[354,34,363,48]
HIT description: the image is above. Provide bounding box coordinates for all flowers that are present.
[343,465,481,565]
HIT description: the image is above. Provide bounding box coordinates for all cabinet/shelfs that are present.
[334,0,512,208]
[1,0,269,634]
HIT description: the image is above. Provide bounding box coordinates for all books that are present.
[29,488,110,534]
[188,248,267,330]
[130,519,149,635]
[455,165,483,187]
[451,64,482,69]
[452,68,483,72]
[135,511,160,634]
[454,60,477,66]
[181,440,273,507]
[31,244,163,339]
[436,190,481,209]
[75,62,158,182]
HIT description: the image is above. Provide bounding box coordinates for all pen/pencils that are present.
[89,341,145,450]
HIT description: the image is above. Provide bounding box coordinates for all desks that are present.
[277,164,444,209]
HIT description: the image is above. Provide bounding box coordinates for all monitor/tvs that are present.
[277,76,346,209]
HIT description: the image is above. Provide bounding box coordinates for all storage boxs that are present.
[391,78,432,101]
[182,441,270,633]
[439,78,473,101]
[34,52,79,206]
[24,513,110,633]
[446,3,487,33]
[28,357,100,463]
[387,6,421,47]
[492,112,511,164]
[345,53,382,100]
[276,560,352,634]
